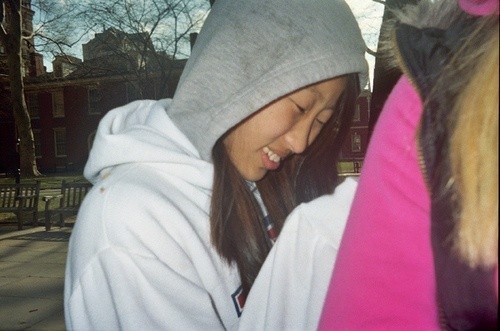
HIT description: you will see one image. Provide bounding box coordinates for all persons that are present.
[237,0,500,331]
[63,0,368,331]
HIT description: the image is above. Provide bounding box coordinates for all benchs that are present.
[0,179,41,230]
[42,180,93,230]
